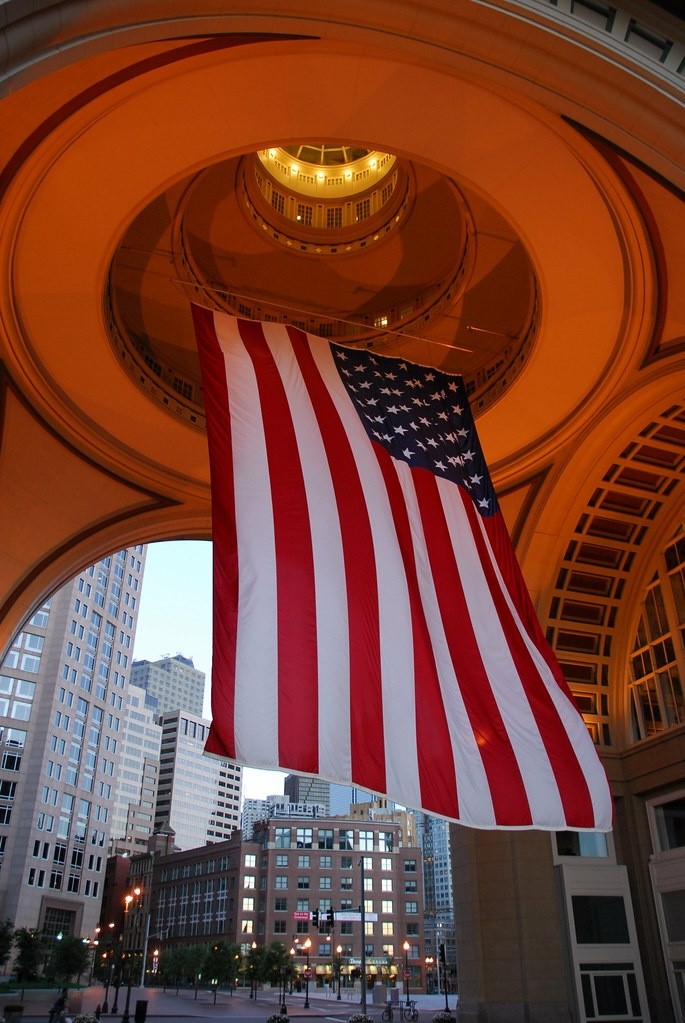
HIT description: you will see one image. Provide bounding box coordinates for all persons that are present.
[49,999,71,1023]
[60,988,68,999]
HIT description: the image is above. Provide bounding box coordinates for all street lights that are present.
[336,945,344,999]
[327,928,336,993]
[304,938,311,1008]
[103,885,141,1023]
[403,941,410,1000]
[426,957,434,995]
[250,941,257,999]
[290,948,295,995]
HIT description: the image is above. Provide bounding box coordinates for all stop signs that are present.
[304,970,312,978]
[405,972,412,980]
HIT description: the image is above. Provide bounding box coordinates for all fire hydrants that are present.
[95,1004,102,1022]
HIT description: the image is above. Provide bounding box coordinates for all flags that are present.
[189,299,616,836]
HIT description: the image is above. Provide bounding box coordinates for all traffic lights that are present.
[330,909,335,927]
[438,945,445,963]
[169,928,172,937]
[312,908,320,929]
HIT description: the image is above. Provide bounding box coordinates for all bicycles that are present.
[402,1000,419,1023]
[381,1001,394,1023]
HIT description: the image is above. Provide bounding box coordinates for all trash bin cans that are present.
[5,1006,23,1023]
[133,999,149,1022]
